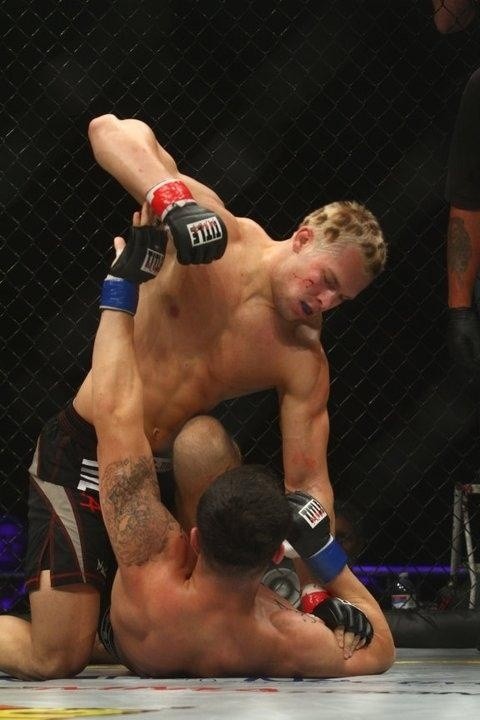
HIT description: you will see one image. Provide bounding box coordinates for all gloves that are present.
[145,177,229,267]
[300,585,374,648]
[282,489,349,586]
[444,306,479,373]
[96,222,169,319]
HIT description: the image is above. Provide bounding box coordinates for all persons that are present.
[329,499,387,613]
[442,66,480,377]
[1,514,30,614]
[0,109,390,685]
[88,201,399,682]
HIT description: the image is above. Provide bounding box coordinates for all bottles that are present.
[391,571,417,610]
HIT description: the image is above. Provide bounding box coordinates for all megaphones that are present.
[261,568,302,611]
